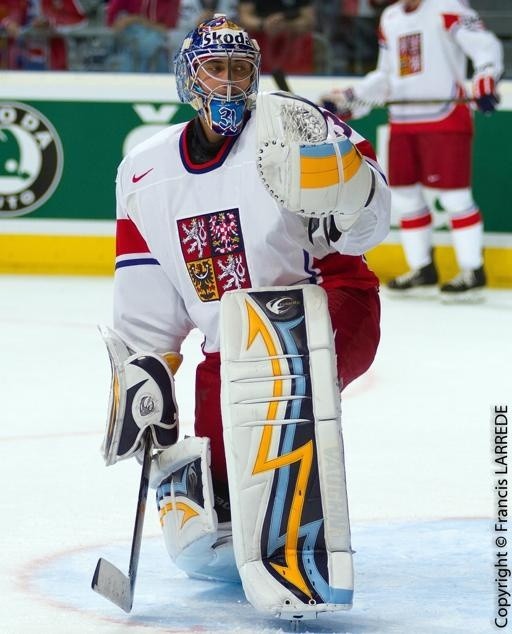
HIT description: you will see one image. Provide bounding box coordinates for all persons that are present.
[99,12,399,627]
[0,0,405,75]
[313,1,504,293]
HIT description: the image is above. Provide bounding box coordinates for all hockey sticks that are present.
[272,65,502,116]
[90,430,154,613]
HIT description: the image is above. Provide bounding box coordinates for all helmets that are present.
[174,16,261,138]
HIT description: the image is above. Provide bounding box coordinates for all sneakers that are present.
[440,268,486,294]
[388,262,438,290]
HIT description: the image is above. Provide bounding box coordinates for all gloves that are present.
[322,86,354,116]
[469,76,500,113]
